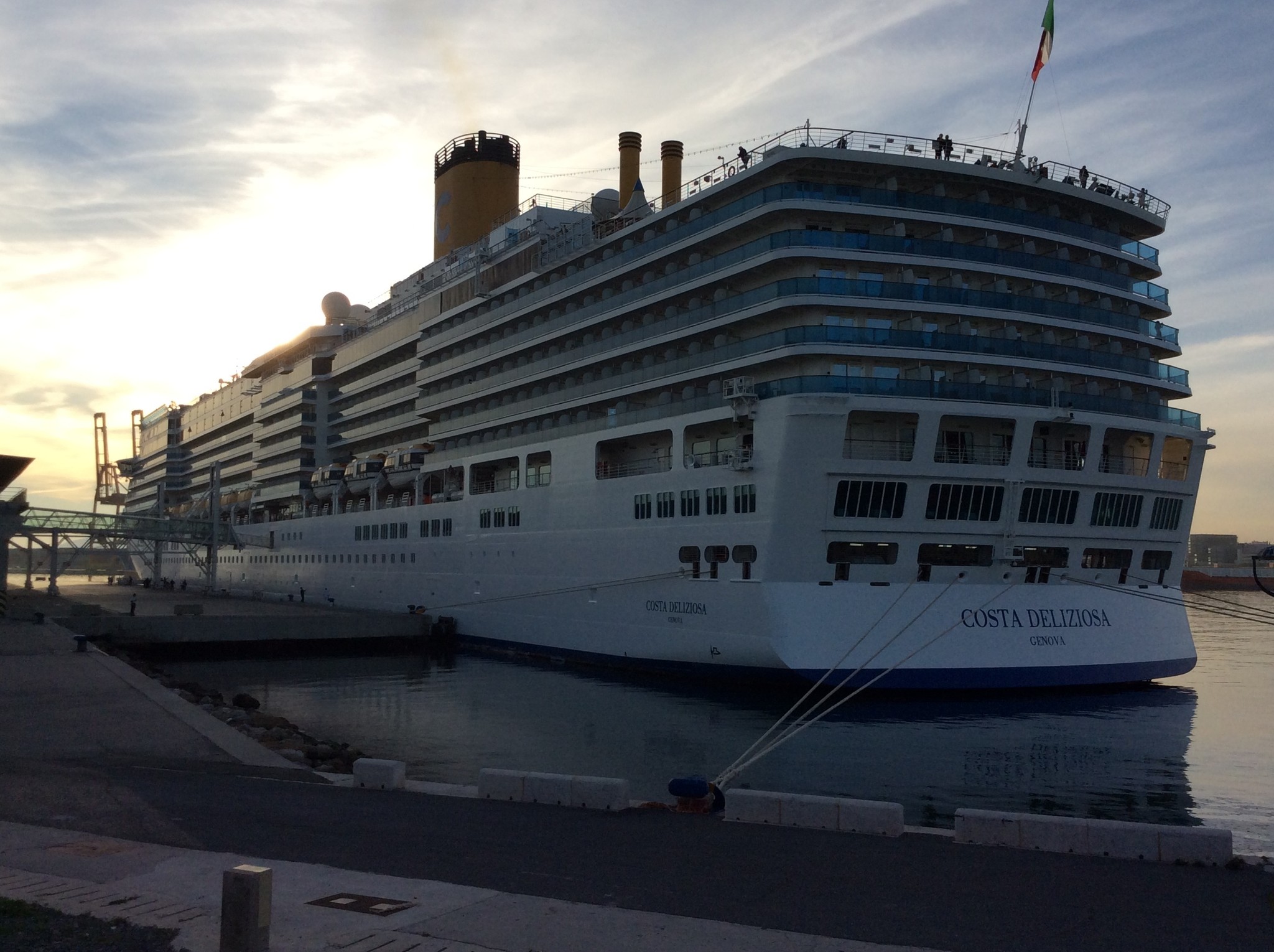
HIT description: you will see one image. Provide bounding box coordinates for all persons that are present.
[1138,188,1145,209]
[943,135,953,161]
[1079,166,1089,189]
[837,137,848,149]
[935,134,944,160]
[737,146,749,169]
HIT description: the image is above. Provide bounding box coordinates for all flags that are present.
[1030,0,1055,81]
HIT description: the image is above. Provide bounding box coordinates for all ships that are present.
[120,1,1216,694]
